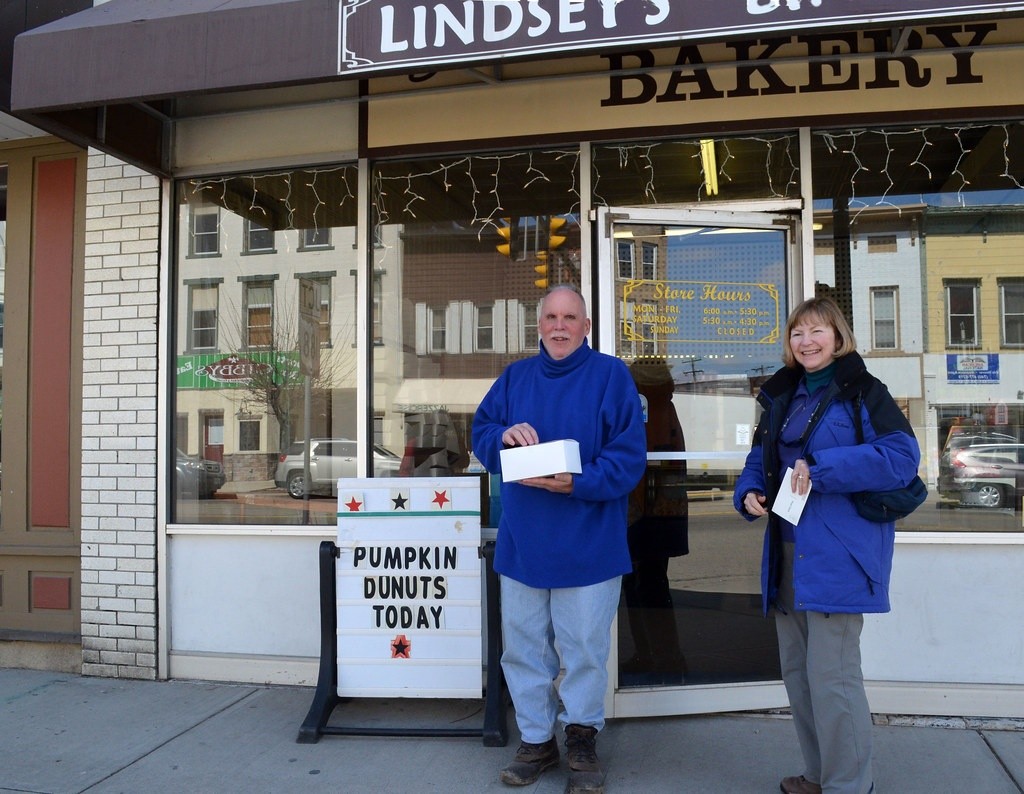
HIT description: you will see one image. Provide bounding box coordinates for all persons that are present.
[730,293,920,794]
[467,285,647,793]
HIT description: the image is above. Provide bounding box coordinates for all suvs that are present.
[274,437,403,499]
[936,425,1024,510]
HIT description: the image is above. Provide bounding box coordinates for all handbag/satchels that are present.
[852,378,928,524]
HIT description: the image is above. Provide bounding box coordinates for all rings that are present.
[798,475,804,480]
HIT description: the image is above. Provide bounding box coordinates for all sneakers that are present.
[501,734,560,785]
[564,723,604,794]
[780,776,824,794]
[627,653,690,685]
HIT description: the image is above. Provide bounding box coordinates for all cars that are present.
[177,447,228,500]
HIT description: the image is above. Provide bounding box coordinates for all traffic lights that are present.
[533,248,555,292]
[543,214,568,254]
[496,216,521,262]
